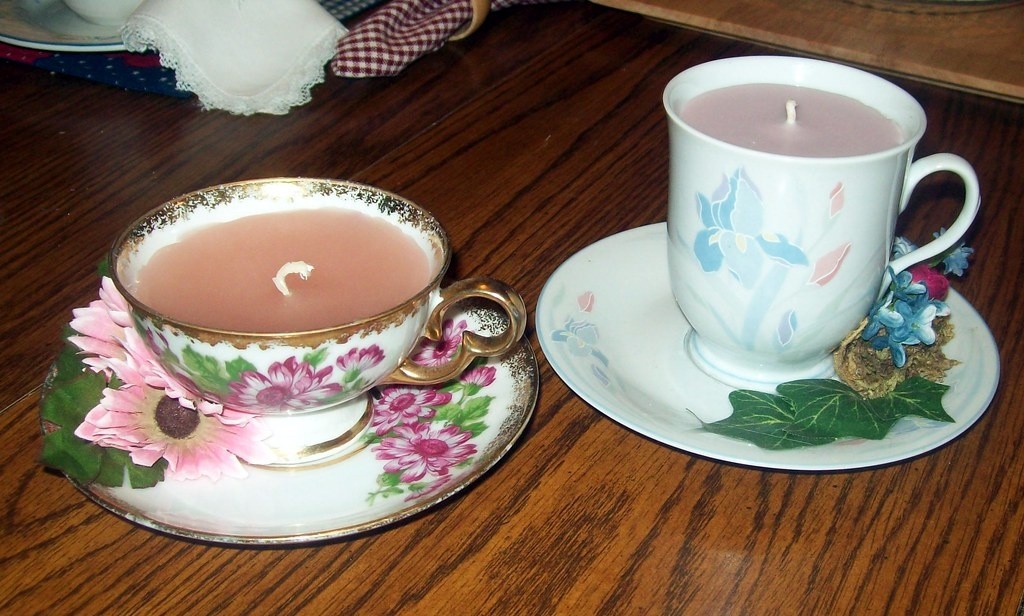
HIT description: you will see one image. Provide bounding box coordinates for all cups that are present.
[662,55,979,392]
[110,178,527,469]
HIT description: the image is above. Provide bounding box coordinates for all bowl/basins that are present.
[60,1,144,27]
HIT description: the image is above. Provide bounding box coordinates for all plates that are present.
[39,283,537,545]
[536,222,1000,472]
[1,2,154,54]
